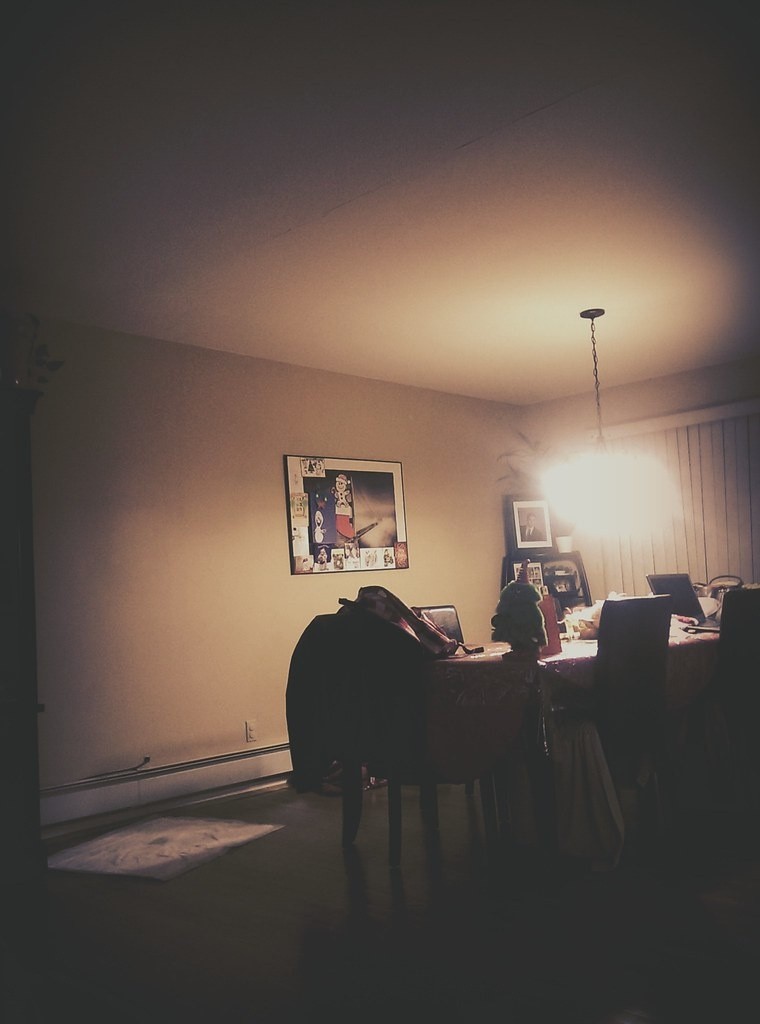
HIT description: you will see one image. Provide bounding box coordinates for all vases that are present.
[555,536,574,553]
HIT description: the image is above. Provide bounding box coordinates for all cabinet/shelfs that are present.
[501,549,593,609]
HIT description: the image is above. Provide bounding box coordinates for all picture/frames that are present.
[505,494,559,554]
[283,454,410,576]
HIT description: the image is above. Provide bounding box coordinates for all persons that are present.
[520,512,543,541]
[286,587,449,788]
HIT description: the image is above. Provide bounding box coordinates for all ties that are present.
[527,529,531,536]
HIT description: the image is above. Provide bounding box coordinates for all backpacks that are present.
[336,586,484,659]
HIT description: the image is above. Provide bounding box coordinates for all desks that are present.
[420,625,721,896]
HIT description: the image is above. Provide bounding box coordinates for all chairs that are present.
[336,589,760,871]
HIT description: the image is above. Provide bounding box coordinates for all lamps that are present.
[541,309,675,536]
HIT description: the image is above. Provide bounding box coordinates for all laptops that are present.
[646,574,721,632]
